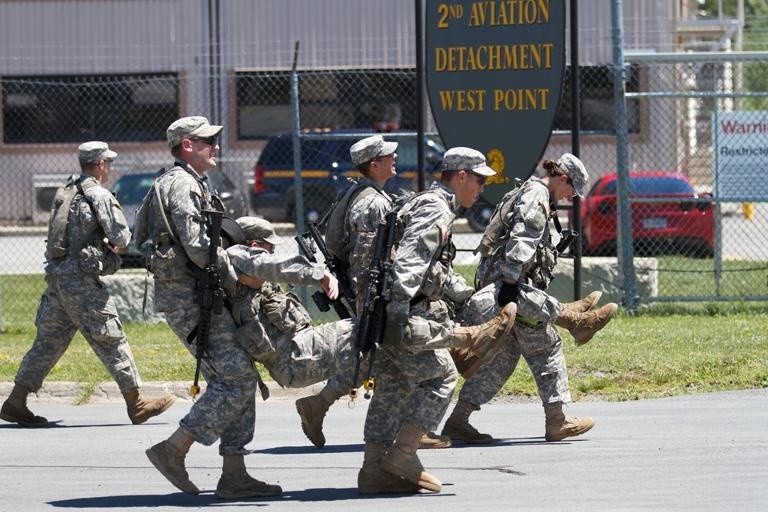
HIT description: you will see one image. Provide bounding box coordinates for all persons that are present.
[389,188,619,346]
[131,116,283,500]
[294,135,400,449]
[439,152,594,443]
[218,216,516,390]
[0,141,176,427]
[357,146,499,495]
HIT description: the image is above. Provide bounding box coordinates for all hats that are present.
[556,152,589,198]
[235,217,283,244]
[441,147,497,177]
[166,116,223,149]
[78,141,118,163]
[349,135,398,166]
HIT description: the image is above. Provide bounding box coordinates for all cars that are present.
[568,172,716,257]
[92,165,246,261]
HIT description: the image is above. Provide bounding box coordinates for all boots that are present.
[145,427,199,495]
[295,385,342,449]
[440,400,492,444]
[0,385,48,427]
[122,387,174,425]
[555,291,618,345]
[545,405,594,442]
[449,302,517,379]
[215,455,282,499]
[358,421,453,494]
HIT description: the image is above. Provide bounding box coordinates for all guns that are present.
[296,220,357,320]
[552,229,580,253]
[350,212,406,407]
[186,209,226,397]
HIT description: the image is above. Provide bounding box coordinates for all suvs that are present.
[246,130,507,233]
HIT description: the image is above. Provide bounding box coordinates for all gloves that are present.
[382,325,404,348]
[497,279,519,307]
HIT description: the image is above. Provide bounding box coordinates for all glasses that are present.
[189,136,214,144]
